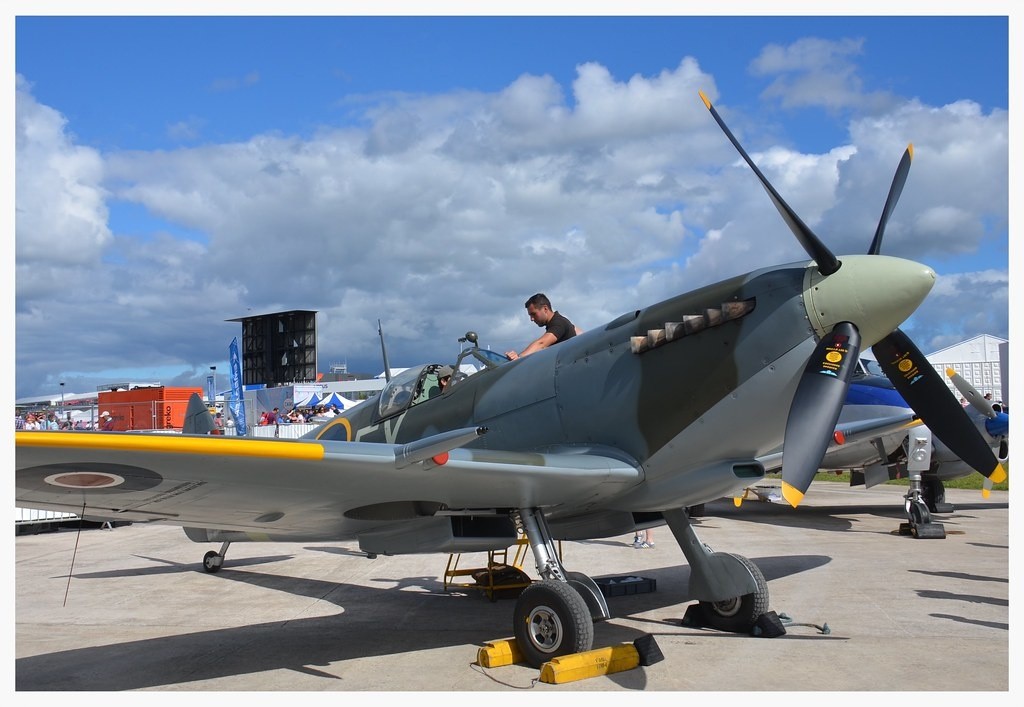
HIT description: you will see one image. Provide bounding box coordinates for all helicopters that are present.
[822,358,1008,512]
[15,85,1009,669]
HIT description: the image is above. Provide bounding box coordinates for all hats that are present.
[295,408,301,412]
[100,411,110,417]
[437,364,468,379]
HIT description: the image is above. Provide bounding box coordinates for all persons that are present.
[100,411,114,431]
[212,413,223,435]
[437,365,468,392]
[15,411,92,431]
[960,398,969,408]
[632,528,655,549]
[227,417,233,428]
[986,393,992,400]
[258,403,341,426]
[505,293,583,361]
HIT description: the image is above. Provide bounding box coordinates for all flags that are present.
[229,337,246,436]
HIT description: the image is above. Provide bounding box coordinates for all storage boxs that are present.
[98,386,203,431]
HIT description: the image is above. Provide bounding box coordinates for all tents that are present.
[293,393,323,410]
[316,392,358,413]
